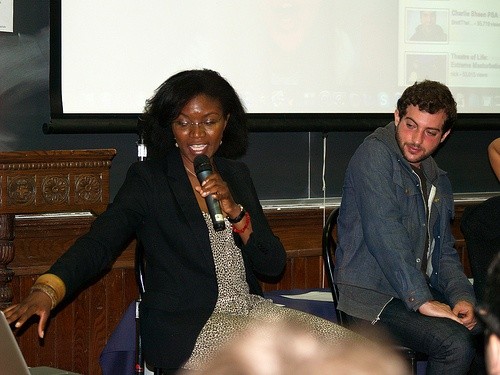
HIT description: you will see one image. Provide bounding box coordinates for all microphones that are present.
[194,155,225,232]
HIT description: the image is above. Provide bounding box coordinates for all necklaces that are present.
[185,164,198,177]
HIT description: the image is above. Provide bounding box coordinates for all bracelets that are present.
[29,273,67,309]
[232,212,250,233]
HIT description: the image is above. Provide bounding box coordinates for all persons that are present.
[333,80,486,375]
[476,138,500,375]
[2,69,387,375]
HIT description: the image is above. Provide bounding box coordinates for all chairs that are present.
[322,205,352,330]
[134,235,166,375]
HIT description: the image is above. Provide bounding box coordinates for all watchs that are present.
[226,204,246,223]
